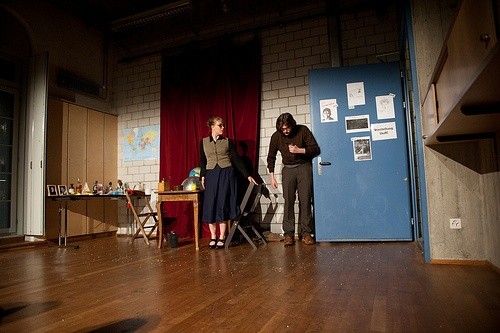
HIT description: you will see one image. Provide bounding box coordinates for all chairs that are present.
[126,190,159,246]
[224,183,267,249]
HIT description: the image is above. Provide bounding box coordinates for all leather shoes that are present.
[284,235,293,245]
[302,234,314,244]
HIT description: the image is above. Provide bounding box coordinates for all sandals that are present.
[208,237,217,249]
[216,236,227,248]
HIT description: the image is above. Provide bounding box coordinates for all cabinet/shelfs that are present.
[25,97,119,243]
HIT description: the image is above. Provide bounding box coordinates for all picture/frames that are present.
[58,185,68,196]
[47,185,58,196]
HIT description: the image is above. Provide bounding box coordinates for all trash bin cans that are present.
[168,231,178,248]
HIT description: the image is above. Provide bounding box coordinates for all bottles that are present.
[76,178,82,194]
[93,180,98,195]
[108,180,123,192]
[69,184,75,194]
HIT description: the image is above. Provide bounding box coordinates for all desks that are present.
[47,195,151,246]
[154,191,203,250]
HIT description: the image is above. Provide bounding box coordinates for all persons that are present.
[200,117,258,249]
[322,108,334,120]
[267,113,321,245]
[50,185,65,195]
